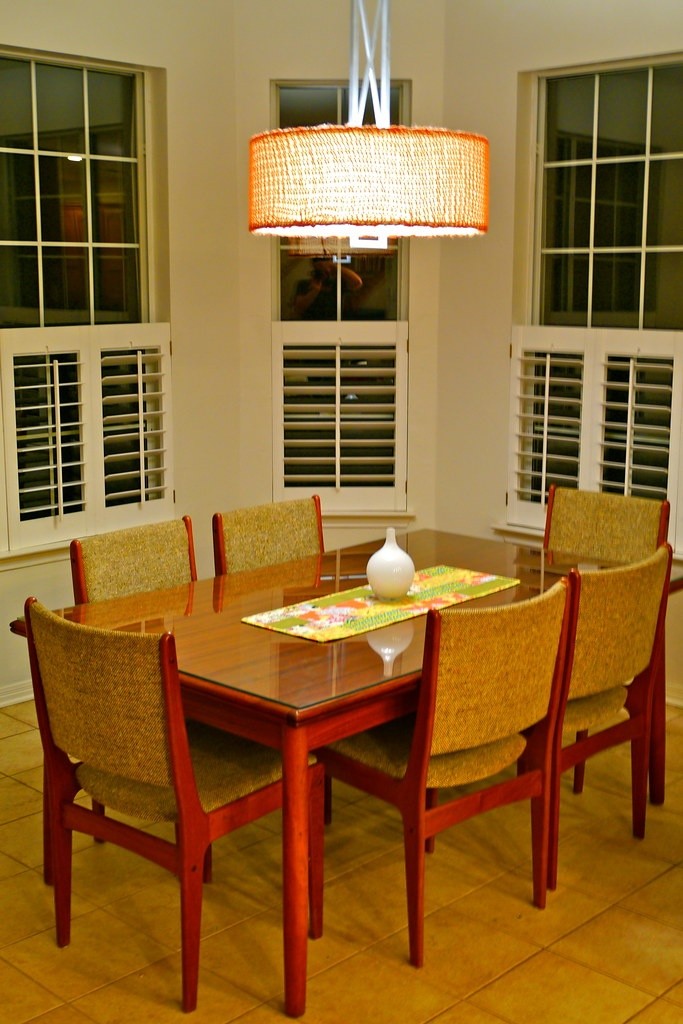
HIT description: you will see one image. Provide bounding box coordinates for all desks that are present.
[6,528,668,1018]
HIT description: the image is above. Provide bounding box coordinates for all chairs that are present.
[68,515,200,609]
[212,495,324,576]
[302,574,571,968]
[429,548,671,891]
[22,597,327,1016]
[541,485,669,793]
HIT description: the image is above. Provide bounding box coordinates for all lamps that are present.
[246,0,490,251]
